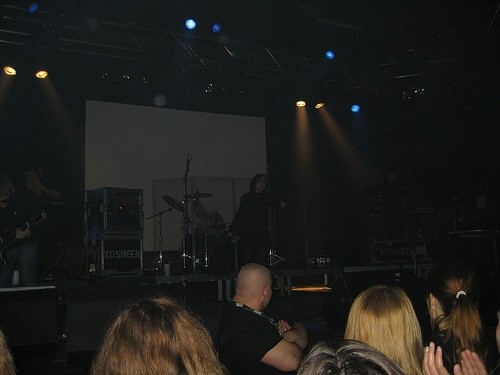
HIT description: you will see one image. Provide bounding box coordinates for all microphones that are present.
[186,156,191,171]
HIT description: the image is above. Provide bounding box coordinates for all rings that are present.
[18,235,22,237]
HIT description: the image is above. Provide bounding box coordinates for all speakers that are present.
[0,293,58,359]
[66,300,128,369]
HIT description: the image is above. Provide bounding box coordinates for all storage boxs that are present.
[88,231,144,275]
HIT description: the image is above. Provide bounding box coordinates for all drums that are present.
[182,231,217,273]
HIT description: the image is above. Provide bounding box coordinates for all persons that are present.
[216,262,308,375]
[226,174,288,273]
[193,202,226,237]
[343,285,424,375]
[421,305,500,375]
[0,173,46,288]
[89,291,232,375]
[423,260,500,375]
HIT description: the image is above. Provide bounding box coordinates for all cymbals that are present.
[162,195,185,213]
[183,190,212,199]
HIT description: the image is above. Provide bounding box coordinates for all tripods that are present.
[178,209,207,274]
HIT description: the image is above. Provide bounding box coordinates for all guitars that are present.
[0,209,48,268]
